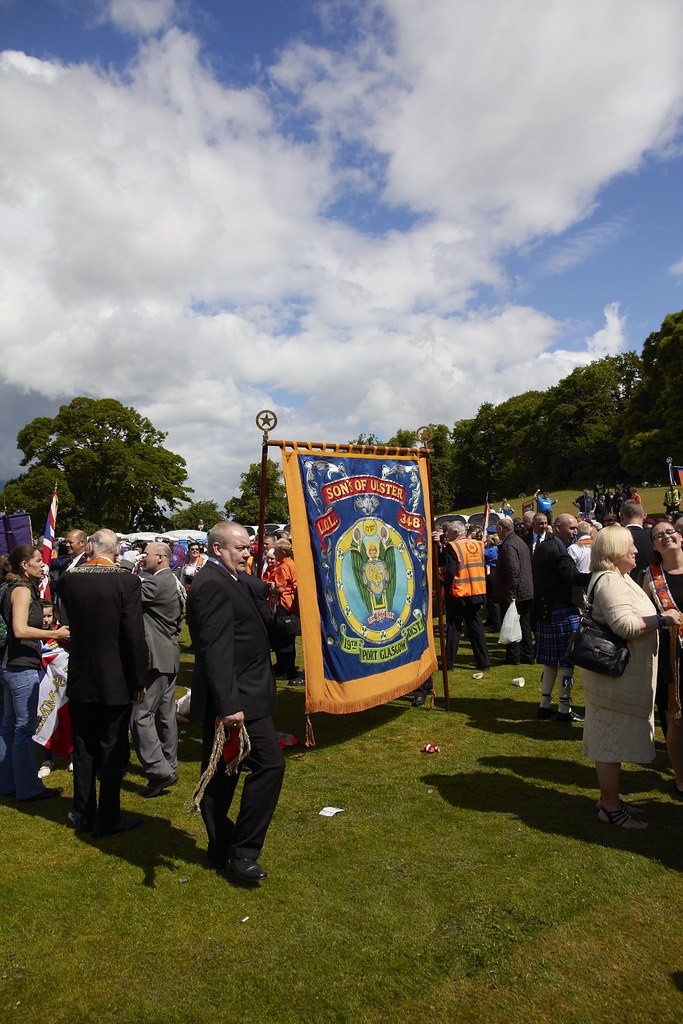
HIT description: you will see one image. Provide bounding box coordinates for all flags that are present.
[36,490,59,602]
[482,499,489,544]
[31,639,73,761]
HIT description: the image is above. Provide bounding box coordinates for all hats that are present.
[486,527,497,534]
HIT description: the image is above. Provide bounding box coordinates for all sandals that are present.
[596,800,650,830]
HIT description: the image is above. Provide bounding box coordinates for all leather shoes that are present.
[139,772,179,799]
[536,707,555,719]
[557,712,585,722]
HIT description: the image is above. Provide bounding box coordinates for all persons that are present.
[193,518,209,533]
[410,482,683,828]
[0,528,303,837]
[186,520,287,879]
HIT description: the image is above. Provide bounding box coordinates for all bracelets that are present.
[656,614,666,630]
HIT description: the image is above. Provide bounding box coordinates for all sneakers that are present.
[69,762,73,771]
[38,761,56,778]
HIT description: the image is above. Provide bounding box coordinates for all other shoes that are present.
[274,672,287,680]
[108,818,140,834]
[439,665,453,671]
[68,818,83,828]
[413,696,426,707]
[288,679,303,686]
[27,788,62,801]
[674,779,683,795]
[476,665,490,671]
[502,660,534,665]
[229,858,267,881]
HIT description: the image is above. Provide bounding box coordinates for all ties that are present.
[536,535,542,546]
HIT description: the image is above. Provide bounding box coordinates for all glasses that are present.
[191,548,199,550]
[141,553,167,559]
[653,529,676,540]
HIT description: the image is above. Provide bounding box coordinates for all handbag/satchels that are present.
[563,572,630,677]
[276,614,300,636]
[498,598,522,644]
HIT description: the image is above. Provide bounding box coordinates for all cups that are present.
[512,677,525,687]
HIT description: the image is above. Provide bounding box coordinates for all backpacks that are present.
[289,585,299,613]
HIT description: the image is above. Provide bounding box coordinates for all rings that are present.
[233,723,237,727]
